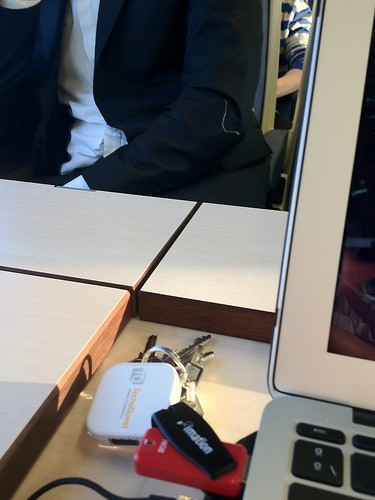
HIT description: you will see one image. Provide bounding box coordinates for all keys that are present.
[130,333,217,418]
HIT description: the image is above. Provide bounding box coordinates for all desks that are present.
[0,177,290,499]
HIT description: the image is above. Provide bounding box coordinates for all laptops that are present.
[241,0,375,500]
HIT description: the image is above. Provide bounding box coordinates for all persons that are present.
[0,0,274,209]
[275,0,313,123]
[326,114,375,361]
